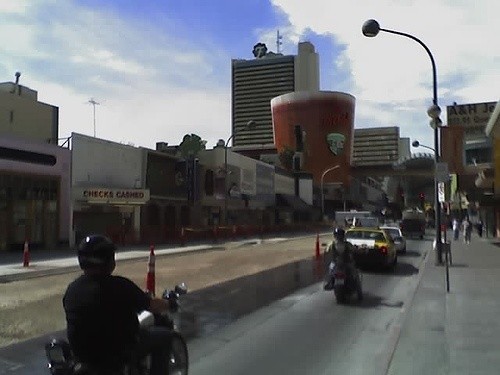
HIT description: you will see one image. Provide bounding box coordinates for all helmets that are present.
[78,234,116,273]
[334,228,345,238]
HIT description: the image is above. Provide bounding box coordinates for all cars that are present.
[341,227,399,270]
[370,226,409,255]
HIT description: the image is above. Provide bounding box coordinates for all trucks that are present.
[333,209,426,244]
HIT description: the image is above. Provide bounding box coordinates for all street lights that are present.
[361,19,444,267]
[321,165,342,216]
[223,121,257,223]
[410,140,452,293]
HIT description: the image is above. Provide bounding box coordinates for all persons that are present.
[324,228,364,299]
[462,216,472,243]
[452,218,460,240]
[62,234,180,375]
[476,216,483,238]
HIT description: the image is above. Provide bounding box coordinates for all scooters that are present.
[45,282,190,375]
[322,243,365,302]
